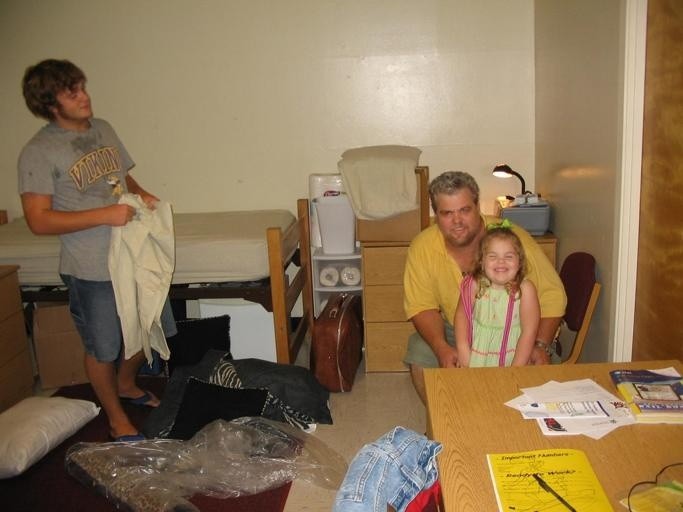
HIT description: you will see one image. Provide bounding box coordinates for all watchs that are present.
[536,340,555,357]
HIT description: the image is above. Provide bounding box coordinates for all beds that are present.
[0,198,316,367]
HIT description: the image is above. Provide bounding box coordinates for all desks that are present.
[425,360,683,512]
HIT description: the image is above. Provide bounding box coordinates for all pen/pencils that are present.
[533,473,576,512]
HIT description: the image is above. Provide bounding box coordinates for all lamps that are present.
[493,163,525,195]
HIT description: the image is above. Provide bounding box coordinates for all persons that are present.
[18,56,160,447]
[402,171,569,439]
[453,229,541,371]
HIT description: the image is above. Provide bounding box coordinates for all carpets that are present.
[0,374,301,512]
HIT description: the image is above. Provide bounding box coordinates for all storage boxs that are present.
[494,197,550,236]
[356,167,431,242]
[31,303,89,389]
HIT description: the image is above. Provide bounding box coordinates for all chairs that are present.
[545,251,602,363]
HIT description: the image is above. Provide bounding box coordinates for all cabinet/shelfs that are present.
[1,265,35,413]
[311,245,364,319]
[360,231,557,373]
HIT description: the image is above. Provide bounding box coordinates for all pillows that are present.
[0,394,102,478]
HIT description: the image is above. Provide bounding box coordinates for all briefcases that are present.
[313,293,364,392]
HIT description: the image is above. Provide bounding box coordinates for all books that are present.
[609,366,682,423]
[486,449,613,512]
[619,479,683,512]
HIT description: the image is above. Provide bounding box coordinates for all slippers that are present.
[119,389,157,408]
[108,427,146,441]
